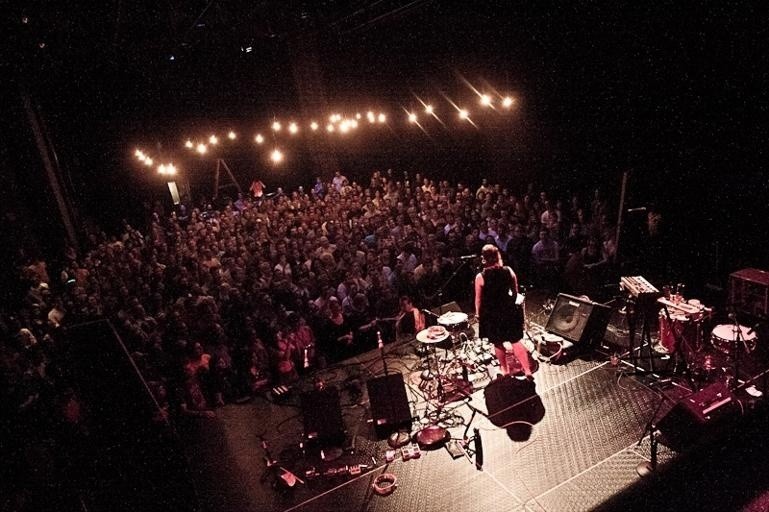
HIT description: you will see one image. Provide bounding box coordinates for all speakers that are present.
[425,301,475,349]
[366,374,413,439]
[652,401,708,455]
[545,292,613,354]
[299,385,342,449]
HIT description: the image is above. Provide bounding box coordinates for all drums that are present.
[658,305,703,352]
[437,311,469,332]
[710,324,758,355]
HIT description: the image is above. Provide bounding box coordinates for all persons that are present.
[647,204,664,240]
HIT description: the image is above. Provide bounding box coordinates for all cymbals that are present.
[416,325,450,343]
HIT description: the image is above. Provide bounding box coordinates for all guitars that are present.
[534,326,563,346]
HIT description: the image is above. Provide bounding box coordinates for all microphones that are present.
[376,330,384,350]
[728,313,739,326]
[461,254,477,259]
[304,345,309,369]
[422,307,440,318]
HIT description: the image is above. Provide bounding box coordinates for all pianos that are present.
[621,275,660,298]
